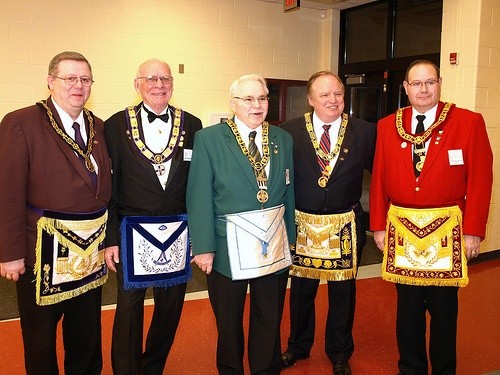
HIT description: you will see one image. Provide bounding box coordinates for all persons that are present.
[101,60,204,375]
[369,58,493,374]
[276,70,377,374]
[0,52,112,375]
[186,73,296,375]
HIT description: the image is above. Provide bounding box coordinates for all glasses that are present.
[54,76,95,87]
[234,97,270,104]
[406,80,439,88]
[137,75,173,85]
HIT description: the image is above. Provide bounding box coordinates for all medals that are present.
[318,177,328,187]
[256,190,268,203]
[416,161,425,172]
[85,160,95,172]
[155,165,166,175]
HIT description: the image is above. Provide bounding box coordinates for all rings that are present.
[5,275,11,279]
[472,250,477,254]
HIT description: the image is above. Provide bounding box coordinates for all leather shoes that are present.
[282,346,309,369]
[333,360,351,375]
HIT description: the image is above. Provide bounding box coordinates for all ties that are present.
[72,122,97,192]
[248,131,267,190]
[412,115,426,178]
[318,125,332,186]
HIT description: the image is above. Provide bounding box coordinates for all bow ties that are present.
[143,104,169,123]
